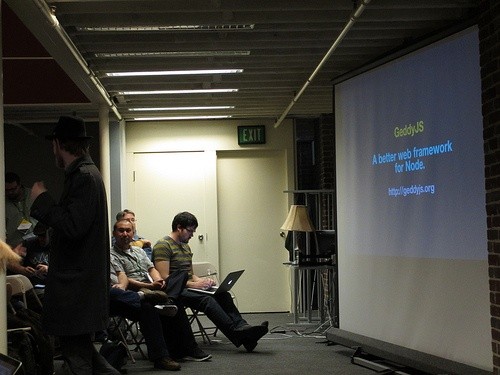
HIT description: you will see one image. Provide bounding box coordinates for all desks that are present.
[283,263,332,327]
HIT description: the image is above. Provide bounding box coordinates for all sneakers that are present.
[154,297,177,317]
[182,349,212,362]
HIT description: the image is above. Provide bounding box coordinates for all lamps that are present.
[279,204,314,261]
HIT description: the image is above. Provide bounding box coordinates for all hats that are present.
[44,116,93,141]
[33,222,48,234]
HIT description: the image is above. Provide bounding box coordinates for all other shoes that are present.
[154,356,180,370]
[243,322,269,352]
[140,288,168,303]
[230,325,268,341]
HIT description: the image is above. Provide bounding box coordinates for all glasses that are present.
[181,226,196,233]
[5,185,18,193]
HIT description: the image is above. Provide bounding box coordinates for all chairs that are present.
[6,263,237,364]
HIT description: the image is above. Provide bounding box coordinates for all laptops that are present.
[187,269,246,296]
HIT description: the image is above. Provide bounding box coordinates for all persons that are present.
[3,173,181,370]
[29,113,121,375]
[112,209,153,261]
[108,219,213,362]
[151,212,269,352]
